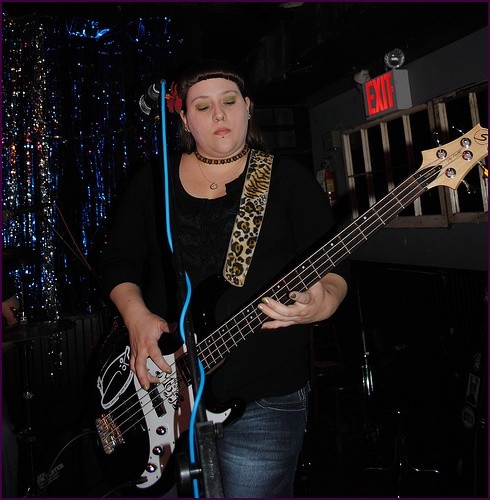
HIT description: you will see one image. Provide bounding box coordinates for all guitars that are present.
[91,124,489,500]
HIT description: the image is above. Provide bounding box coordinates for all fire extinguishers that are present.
[324,160,338,208]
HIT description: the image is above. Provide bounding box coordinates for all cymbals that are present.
[2,246,37,272]
[2,318,78,344]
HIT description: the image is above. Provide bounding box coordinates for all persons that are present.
[103,56,358,500]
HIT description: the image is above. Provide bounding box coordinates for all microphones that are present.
[139,79,166,116]
[2,202,52,222]
